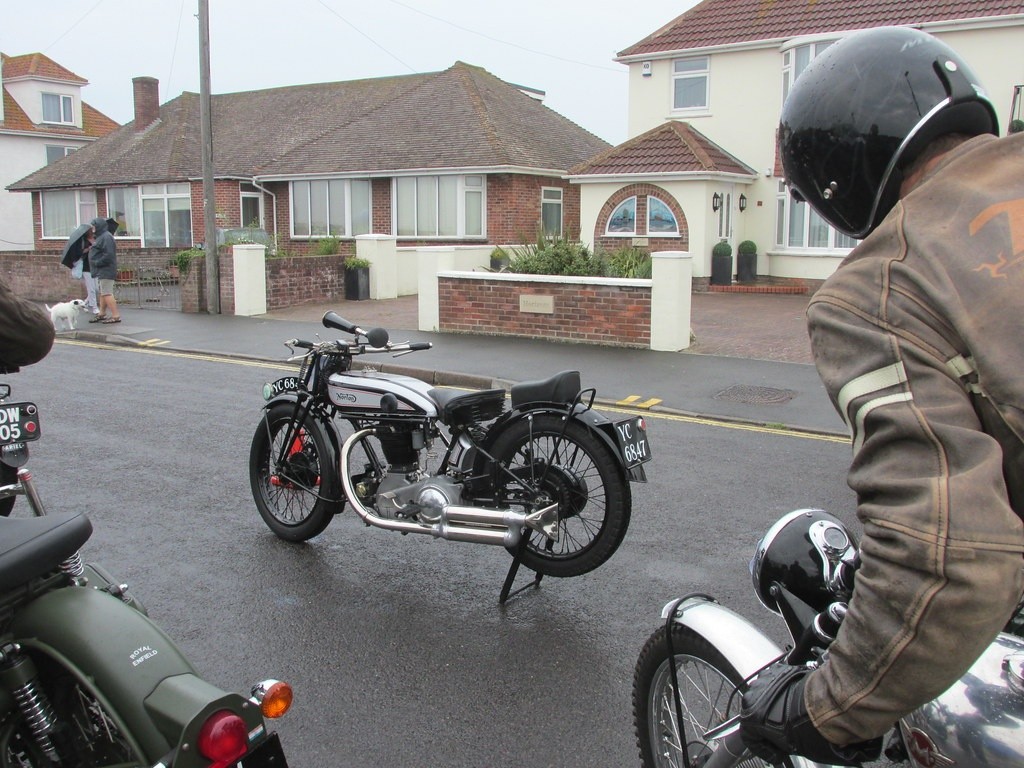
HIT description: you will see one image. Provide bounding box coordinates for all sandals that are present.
[102,316,122,324]
[89,314,107,323]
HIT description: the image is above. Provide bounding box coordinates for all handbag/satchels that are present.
[72,258,83,279]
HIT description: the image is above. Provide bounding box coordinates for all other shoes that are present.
[92,309,100,314]
[84,307,89,312]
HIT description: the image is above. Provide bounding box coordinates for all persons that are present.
[75,217,122,325]
[0,276,55,366]
[739,27,1024,768]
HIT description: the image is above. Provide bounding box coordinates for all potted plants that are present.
[118,267,132,279]
[490,247,510,270]
[738,240,758,284]
[710,241,733,287]
[164,259,180,277]
[342,257,369,302]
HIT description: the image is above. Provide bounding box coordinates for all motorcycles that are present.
[250,309,654,601]
[1,377,297,768]
[631,503,1024,768]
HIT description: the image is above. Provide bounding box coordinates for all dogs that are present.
[44,299,89,332]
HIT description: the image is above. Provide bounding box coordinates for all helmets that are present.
[779,27,1000,238]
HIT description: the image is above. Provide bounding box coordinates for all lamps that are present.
[713,193,721,212]
[740,193,748,212]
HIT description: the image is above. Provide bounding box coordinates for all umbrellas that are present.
[60,218,119,269]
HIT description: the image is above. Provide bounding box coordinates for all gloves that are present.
[739,664,884,765]
